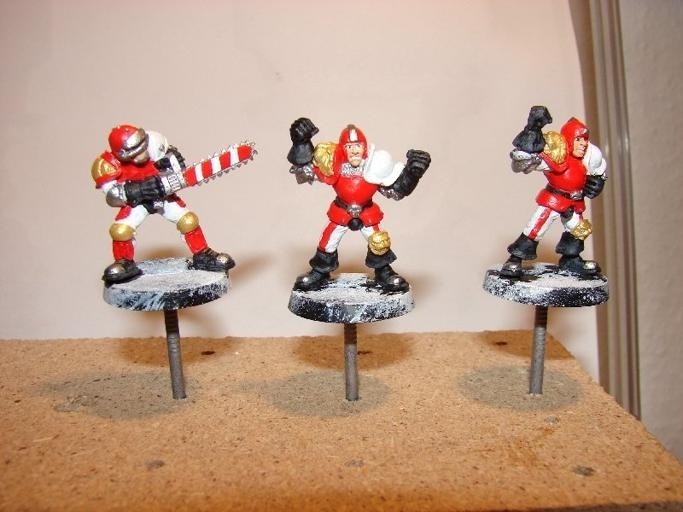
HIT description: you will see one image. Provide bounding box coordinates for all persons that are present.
[285,116,432,295]
[89,122,258,284]
[497,105,611,280]
